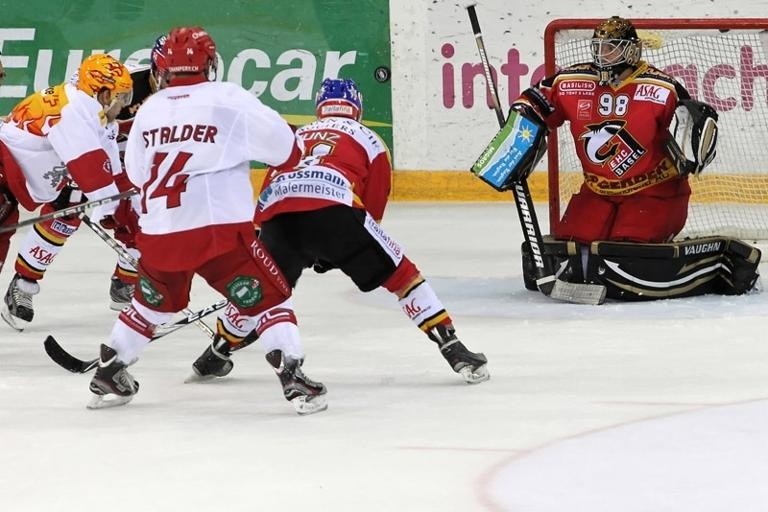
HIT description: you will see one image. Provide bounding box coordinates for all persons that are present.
[1,51,135,322]
[470,13,761,306]
[191,75,487,378]
[88,23,328,401]
[3,42,158,323]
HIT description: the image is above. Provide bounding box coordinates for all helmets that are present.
[77,54,134,107]
[590,16,641,71]
[315,78,362,120]
[154,27,216,80]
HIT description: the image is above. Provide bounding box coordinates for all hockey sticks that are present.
[44,299,231,373]
[464,0,608,305]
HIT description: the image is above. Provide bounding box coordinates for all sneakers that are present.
[429,325,486,371]
[193,335,233,375]
[110,278,135,302]
[266,350,327,401]
[4,274,39,322]
[90,344,139,395]
